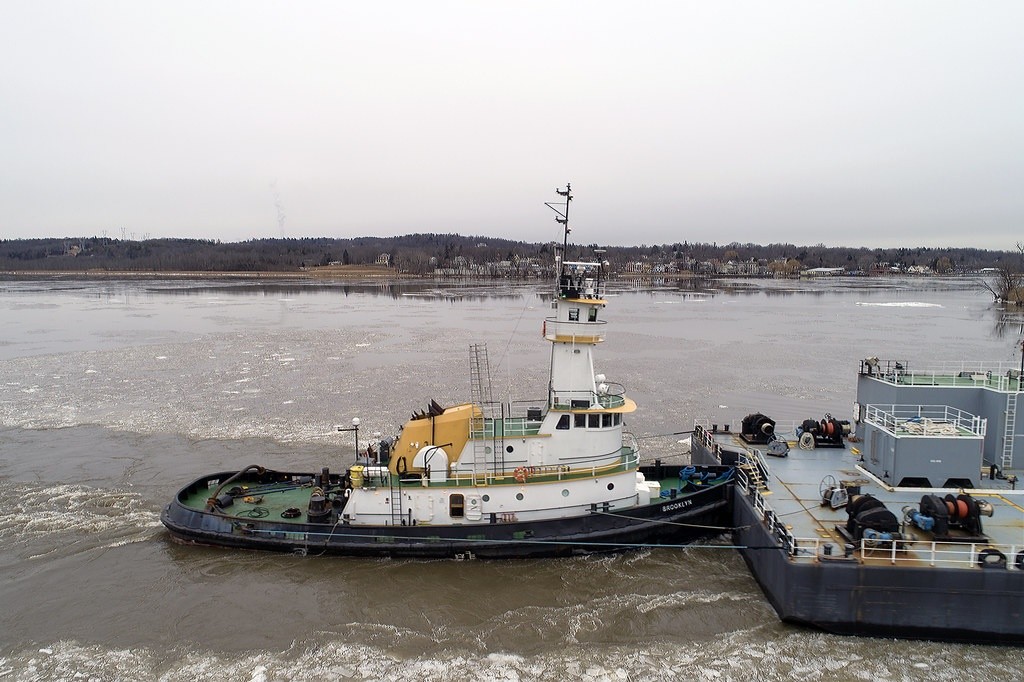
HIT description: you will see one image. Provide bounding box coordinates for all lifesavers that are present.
[514,467,528,481]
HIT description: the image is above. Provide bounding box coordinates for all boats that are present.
[160,178,737,559]
